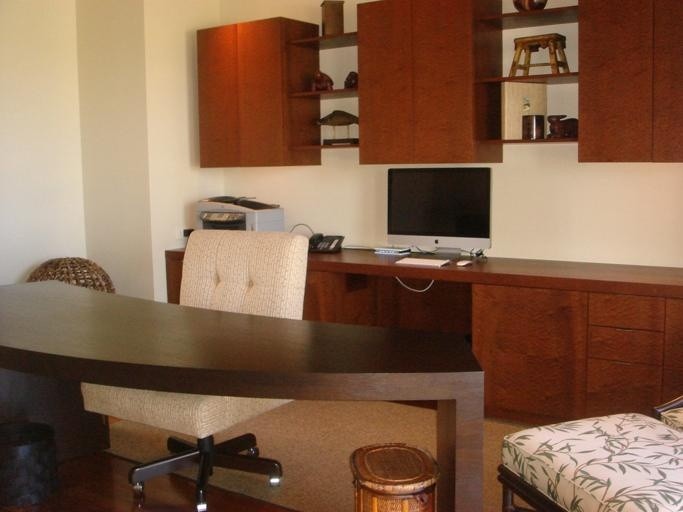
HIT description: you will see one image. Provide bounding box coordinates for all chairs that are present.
[128,229,309,512]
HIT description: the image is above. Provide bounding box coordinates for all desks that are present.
[0,279,485,512]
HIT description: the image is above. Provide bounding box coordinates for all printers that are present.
[194,196,285,236]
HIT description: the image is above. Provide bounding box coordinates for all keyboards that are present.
[395,257,449,268]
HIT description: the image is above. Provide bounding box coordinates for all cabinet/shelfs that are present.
[585,263,663,420]
[197,17,322,169]
[577,0,683,164]
[503,6,580,144]
[357,0,502,165]
[291,1,360,149]
[165,248,323,323]
[664,266,683,402]
[470,257,586,426]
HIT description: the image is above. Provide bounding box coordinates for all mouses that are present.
[456,258,474,267]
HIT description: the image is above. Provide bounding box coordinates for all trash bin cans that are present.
[0,421,54,506]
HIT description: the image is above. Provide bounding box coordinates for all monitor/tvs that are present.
[387,167,494,251]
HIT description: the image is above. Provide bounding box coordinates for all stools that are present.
[496,411,683,512]
[350,442,439,512]
[655,394,683,435]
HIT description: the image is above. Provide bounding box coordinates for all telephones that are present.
[308,233,344,253]
